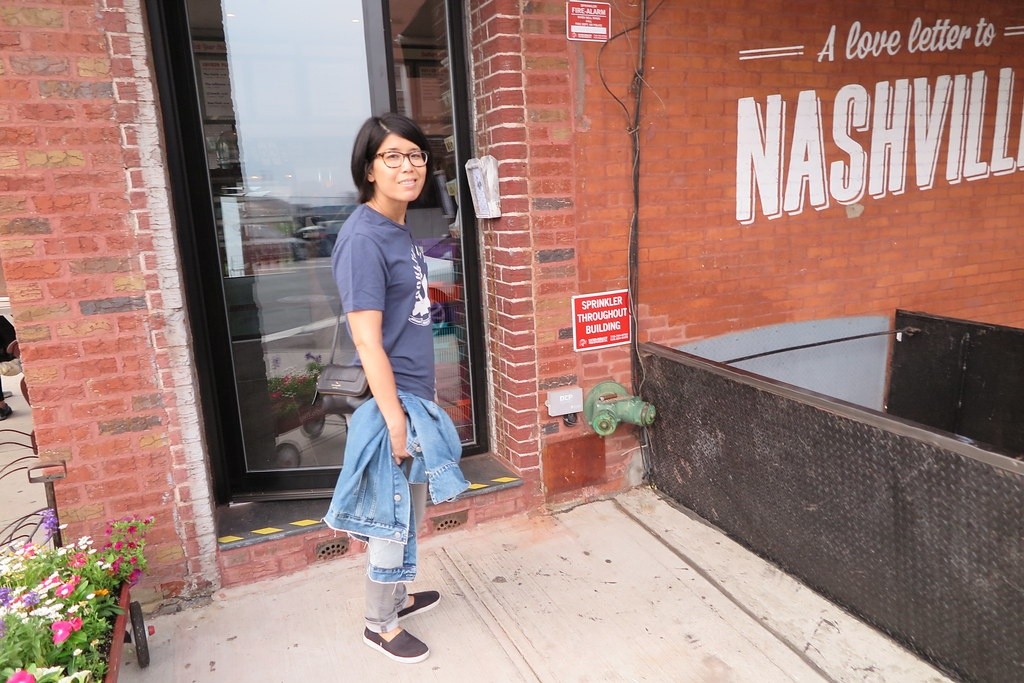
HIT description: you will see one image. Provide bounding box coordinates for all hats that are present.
[7,340,17,353]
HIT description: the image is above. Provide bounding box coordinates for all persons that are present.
[323,114,471,663]
[0,315,23,421]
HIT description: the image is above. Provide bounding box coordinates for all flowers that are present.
[0,508,158,683]
[267,351,324,417]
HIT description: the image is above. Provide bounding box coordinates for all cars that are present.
[420,232,455,336]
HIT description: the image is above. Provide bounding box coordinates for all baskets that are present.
[415,233,476,441]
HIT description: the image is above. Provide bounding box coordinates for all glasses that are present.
[374,151,430,169]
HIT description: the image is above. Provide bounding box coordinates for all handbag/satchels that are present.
[311,363,374,414]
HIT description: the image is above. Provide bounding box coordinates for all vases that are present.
[276,400,326,433]
[97,581,131,683]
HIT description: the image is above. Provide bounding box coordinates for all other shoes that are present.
[0,403,12,420]
[397,591,441,620]
[363,625,429,663]
